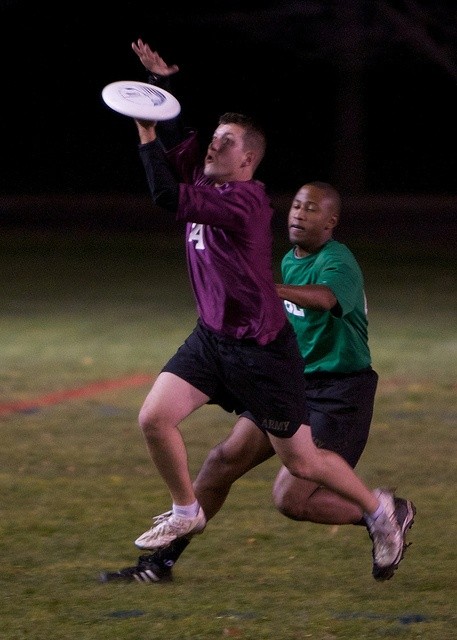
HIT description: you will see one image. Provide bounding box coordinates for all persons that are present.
[95,186,408,583]
[128,35,419,583]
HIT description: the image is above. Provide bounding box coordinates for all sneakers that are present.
[369,487,404,569]
[135,505,207,551]
[371,498,416,583]
[99,561,173,585]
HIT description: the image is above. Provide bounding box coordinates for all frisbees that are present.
[102,81,181,120]
